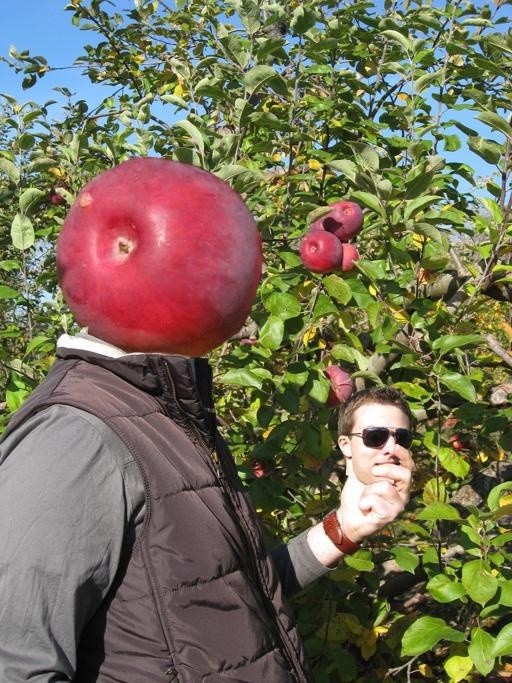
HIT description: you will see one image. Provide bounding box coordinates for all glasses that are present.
[341,426,413,450]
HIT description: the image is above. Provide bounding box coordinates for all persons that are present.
[0,327,415,682]
[336,384,414,487]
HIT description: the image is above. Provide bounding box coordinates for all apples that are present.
[441,420,463,451]
[336,242,359,273]
[299,232,342,274]
[323,366,352,407]
[315,201,363,240]
[249,462,275,479]
[58,158,260,359]
[49,185,69,205]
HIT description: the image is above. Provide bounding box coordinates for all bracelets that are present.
[322,508,361,555]
